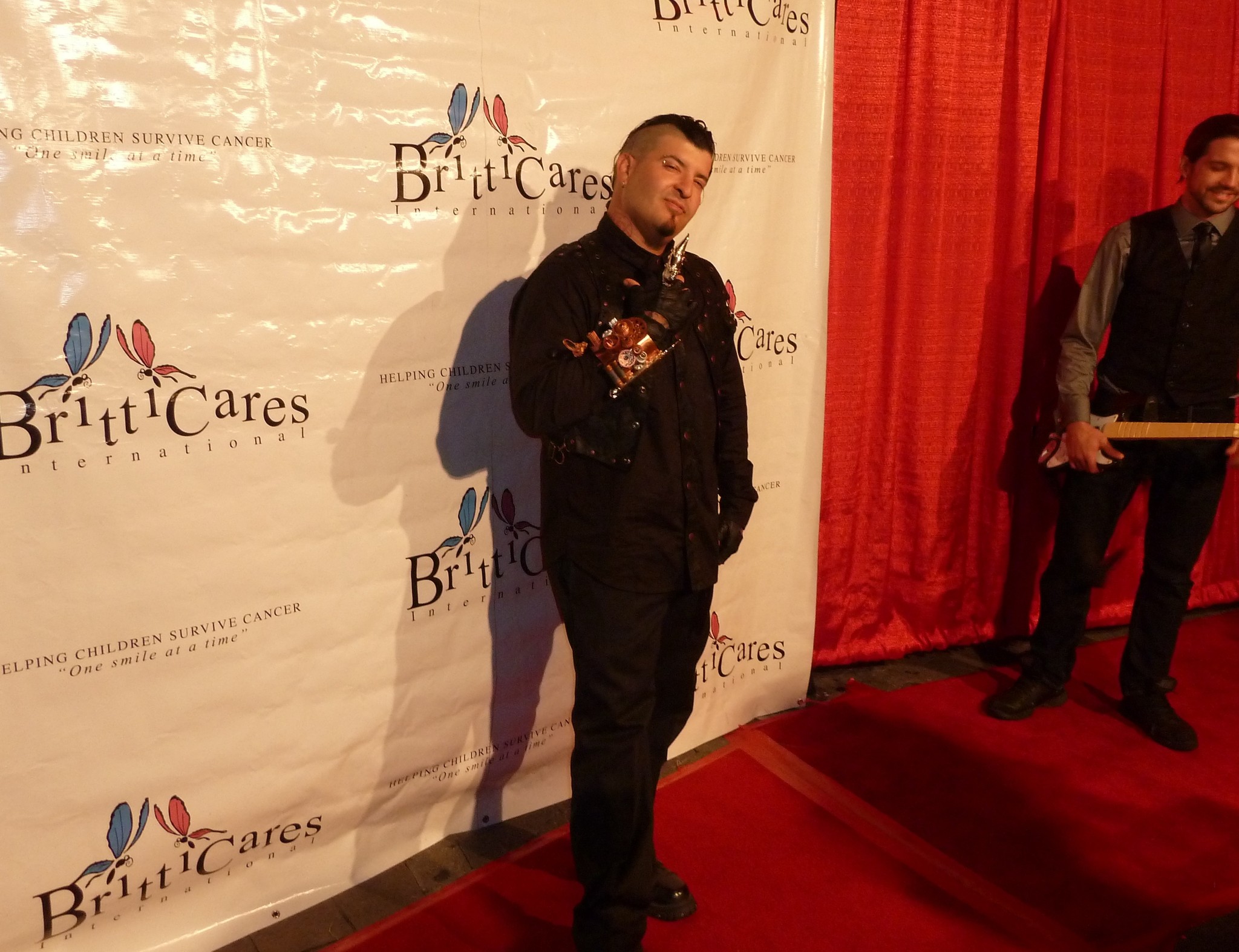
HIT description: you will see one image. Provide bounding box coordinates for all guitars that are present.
[1038,405,1239,470]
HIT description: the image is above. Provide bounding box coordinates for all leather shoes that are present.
[646,861,696,921]
[991,667,1068,720]
[1117,691,1198,752]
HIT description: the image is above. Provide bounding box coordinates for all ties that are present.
[1192,222,1214,265]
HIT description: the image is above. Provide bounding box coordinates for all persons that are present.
[509,114,759,951]
[983,111,1238,750]
